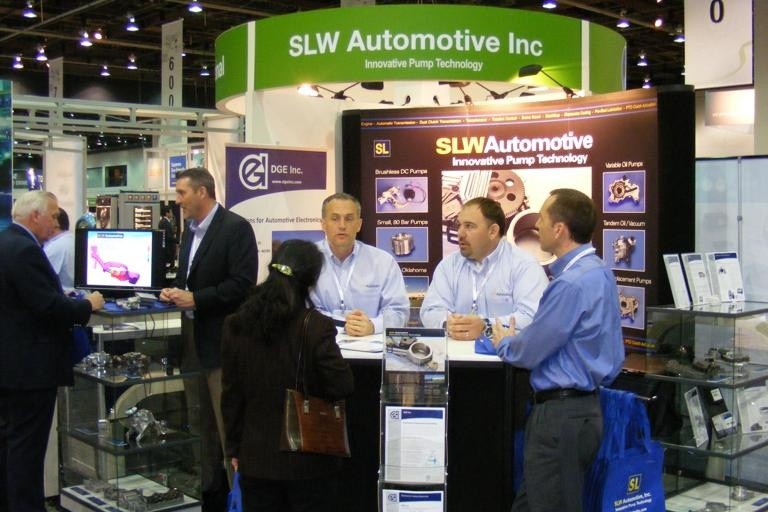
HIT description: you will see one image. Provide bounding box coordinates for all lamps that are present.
[12,0,210,76]
[296,79,472,105]
[543,0,685,89]
[519,64,577,99]
[499,84,541,100]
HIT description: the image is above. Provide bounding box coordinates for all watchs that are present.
[480,317,493,340]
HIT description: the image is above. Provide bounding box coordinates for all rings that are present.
[464,331,469,338]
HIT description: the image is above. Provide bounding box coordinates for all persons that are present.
[110,167,127,186]
[158,206,177,272]
[43,206,75,300]
[297,191,409,472]
[1,188,105,510]
[489,187,623,510]
[219,237,356,511]
[417,195,550,343]
[161,167,260,494]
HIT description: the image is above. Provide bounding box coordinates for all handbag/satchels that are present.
[280,388,353,457]
[513,430,666,512]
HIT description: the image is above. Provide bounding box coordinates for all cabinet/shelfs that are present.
[643,301,768,512]
[57,307,203,512]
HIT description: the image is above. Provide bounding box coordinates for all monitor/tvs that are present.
[74,227,167,299]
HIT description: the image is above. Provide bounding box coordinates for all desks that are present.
[338,333,528,512]
